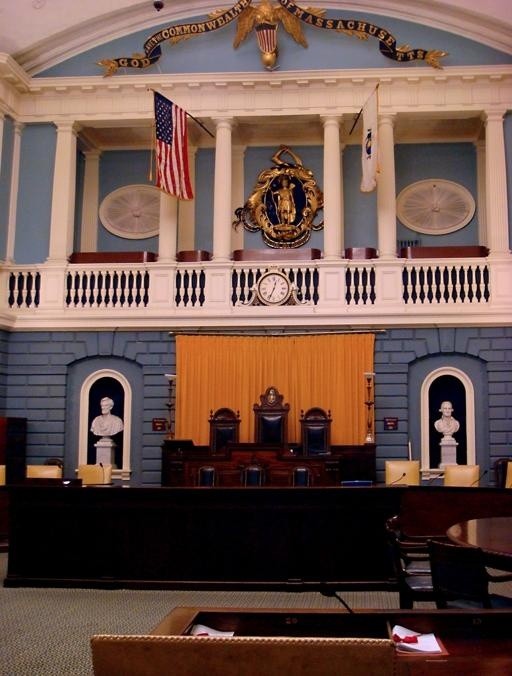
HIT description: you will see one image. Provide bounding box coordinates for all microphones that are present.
[99,462,104,486]
[58,464,63,485]
[318,581,356,615]
[388,473,406,487]
[470,469,488,487]
[427,473,445,486]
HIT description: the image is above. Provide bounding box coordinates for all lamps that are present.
[165,374,177,440]
[364,372,375,444]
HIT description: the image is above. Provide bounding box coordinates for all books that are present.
[344,479,373,489]
[391,625,449,657]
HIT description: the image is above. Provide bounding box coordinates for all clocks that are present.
[257,271,292,306]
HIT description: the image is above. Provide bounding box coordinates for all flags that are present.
[152,90,192,200]
[361,89,379,194]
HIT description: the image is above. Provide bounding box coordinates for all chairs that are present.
[426,540,511,608]
[90,634,397,676]
[385,514,448,610]
[245,464,262,486]
[292,466,310,488]
[198,465,214,487]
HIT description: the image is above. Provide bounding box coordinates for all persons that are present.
[434,399,462,435]
[275,177,298,223]
[90,395,122,437]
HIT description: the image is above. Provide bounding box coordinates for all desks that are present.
[447,517,512,573]
